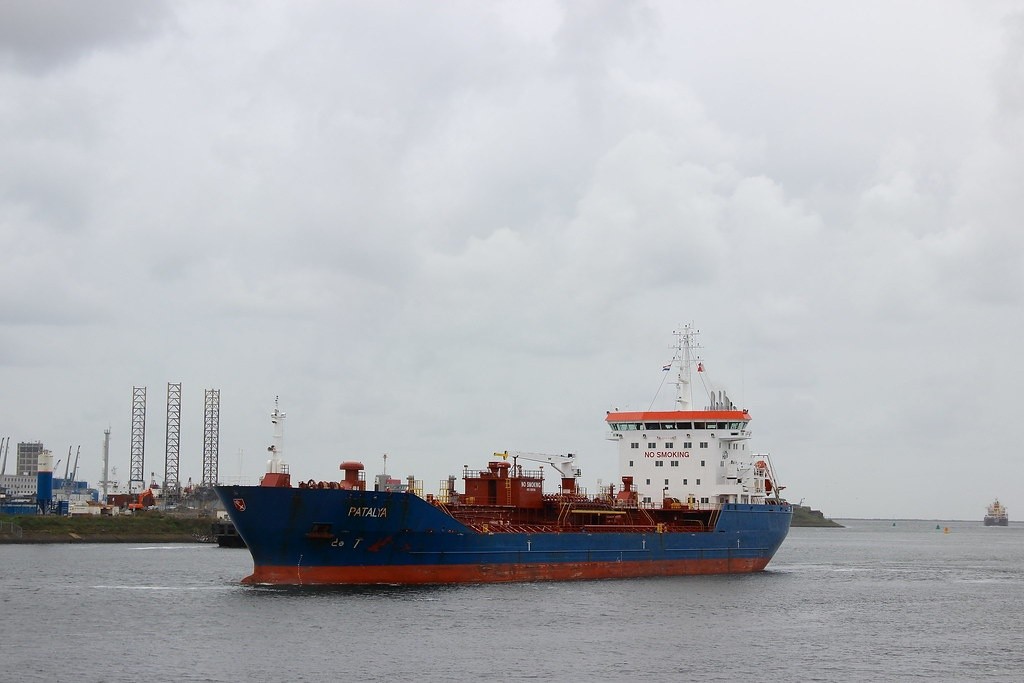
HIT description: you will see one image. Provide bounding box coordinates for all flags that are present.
[661,364,670,371]
[698,362,704,372]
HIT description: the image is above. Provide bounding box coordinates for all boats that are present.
[984,497,1008,526]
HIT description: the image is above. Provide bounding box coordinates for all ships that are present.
[214,324,796,587]
